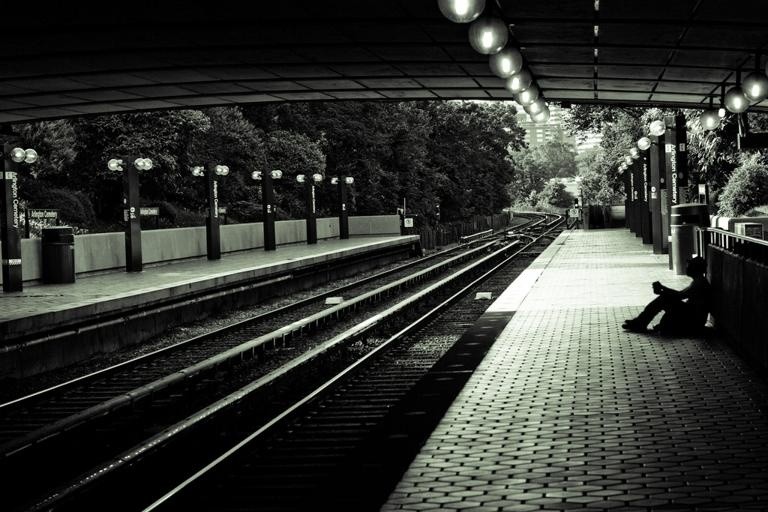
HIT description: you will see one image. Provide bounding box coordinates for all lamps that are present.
[701,51,768,131]
[439,0,550,123]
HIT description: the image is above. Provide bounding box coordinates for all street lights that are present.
[192,164,229,261]
[330,175,355,239]
[618,115,704,255]
[252,168,283,251]
[0,143,38,294]
[296,173,322,245]
[107,156,153,272]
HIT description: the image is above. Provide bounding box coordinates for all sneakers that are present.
[622,322,647,333]
[625,318,637,323]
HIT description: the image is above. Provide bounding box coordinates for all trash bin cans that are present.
[41,227,75,284]
[669,202,710,275]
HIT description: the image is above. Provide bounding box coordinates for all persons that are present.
[622,257,711,340]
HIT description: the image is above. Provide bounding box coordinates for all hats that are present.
[685,256,708,275]
